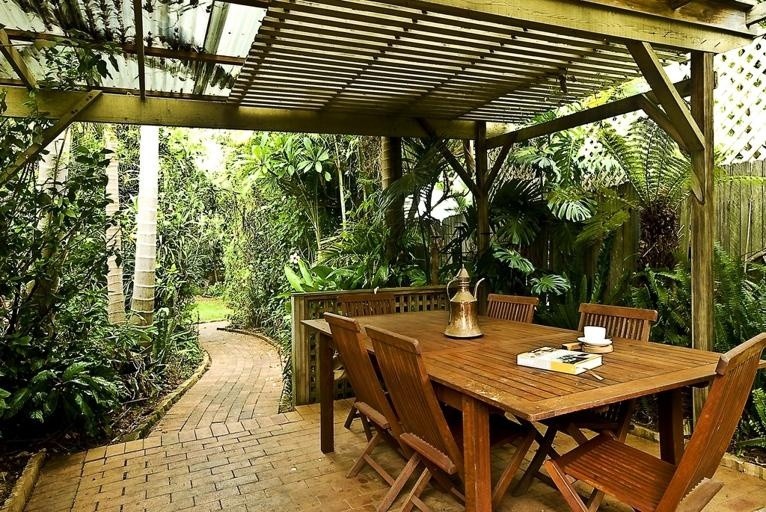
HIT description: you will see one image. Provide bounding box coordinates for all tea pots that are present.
[445,262,487,338]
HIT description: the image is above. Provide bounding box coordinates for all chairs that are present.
[510,299,660,512]
[362,320,537,512]
[485,294,540,324]
[339,291,398,440]
[326,310,417,510]
[541,326,766,512]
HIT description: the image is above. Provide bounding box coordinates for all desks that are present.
[297,304,766,511]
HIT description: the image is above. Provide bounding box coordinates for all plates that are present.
[576,336,614,345]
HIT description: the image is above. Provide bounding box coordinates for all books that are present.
[514,345,603,377]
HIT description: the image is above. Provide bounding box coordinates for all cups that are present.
[585,326,606,340]
